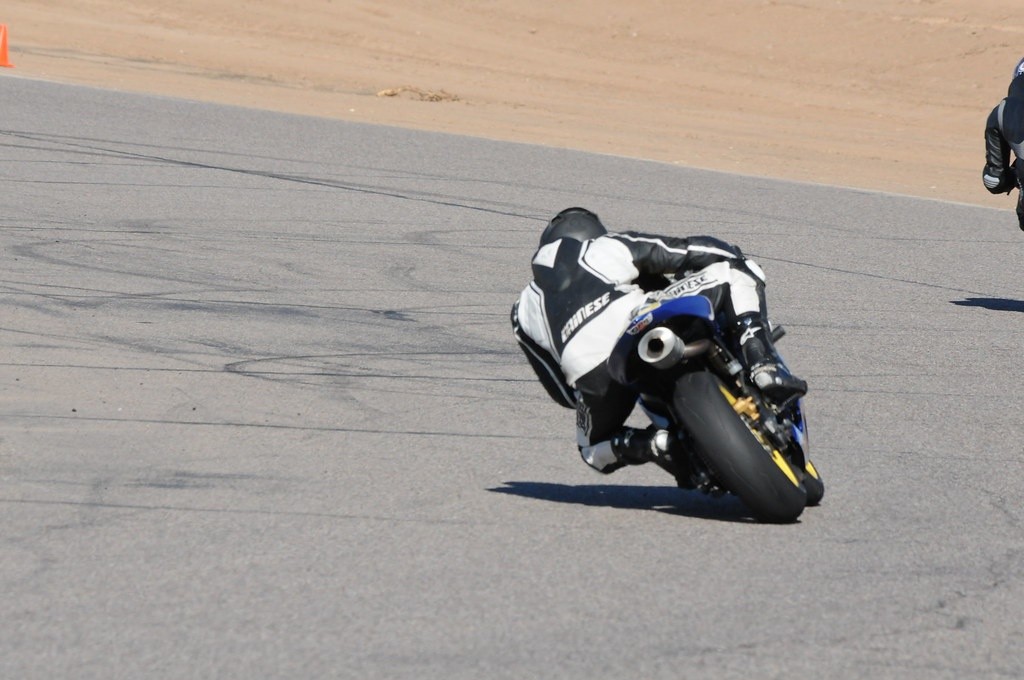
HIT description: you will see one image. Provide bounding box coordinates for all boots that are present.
[608,425,694,468]
[735,311,808,403]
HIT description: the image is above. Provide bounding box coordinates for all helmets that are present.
[539,207,607,246]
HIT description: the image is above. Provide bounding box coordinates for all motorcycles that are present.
[609,245,824,525]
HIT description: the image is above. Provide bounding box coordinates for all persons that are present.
[510,206,807,476]
[983,61,1024,234]
